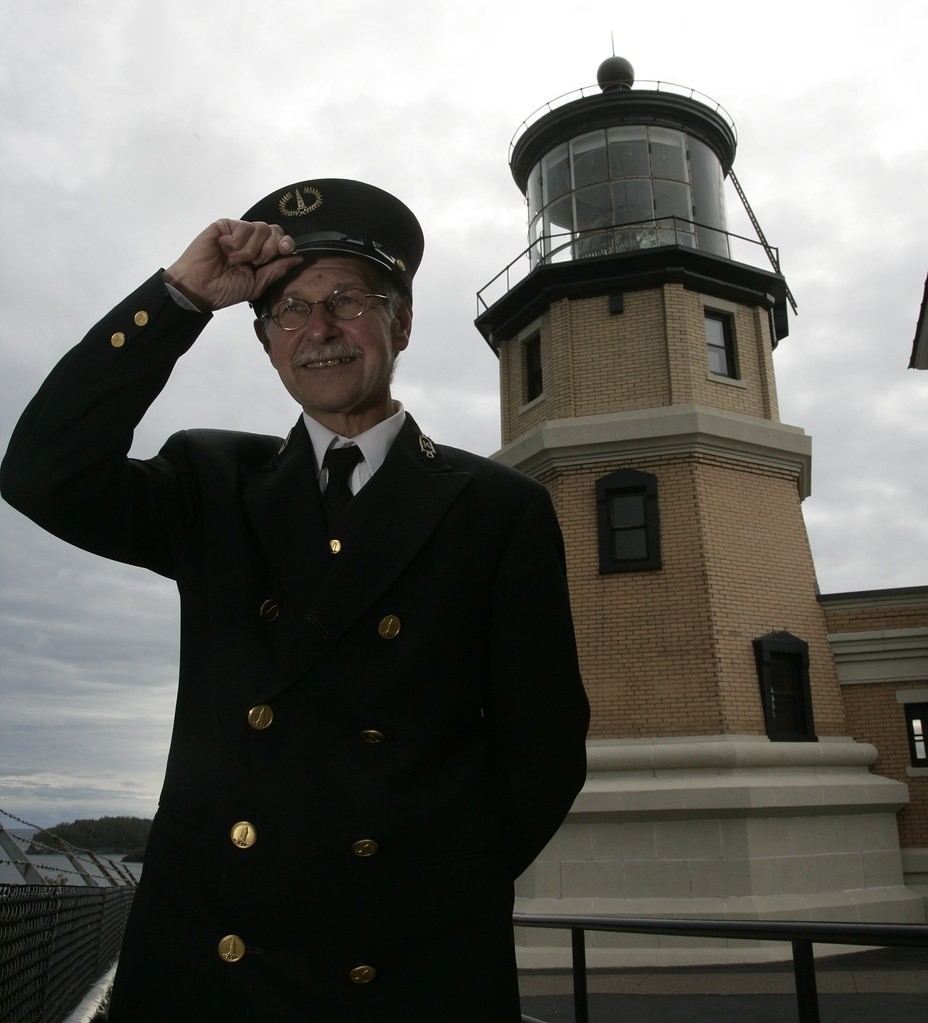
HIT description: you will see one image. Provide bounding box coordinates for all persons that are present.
[1,179,591,1023]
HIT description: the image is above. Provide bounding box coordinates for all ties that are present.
[321,444,365,499]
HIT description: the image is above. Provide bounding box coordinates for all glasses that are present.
[258,287,390,331]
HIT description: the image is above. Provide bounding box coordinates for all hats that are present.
[239,179,425,297]
